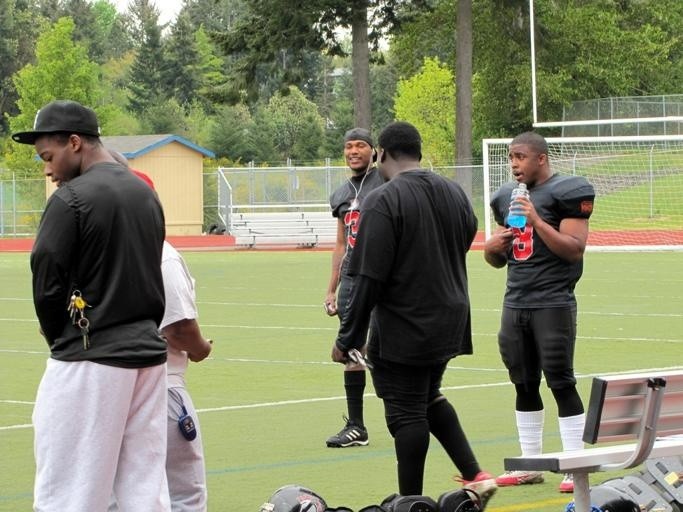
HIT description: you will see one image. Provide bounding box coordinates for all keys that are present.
[67,295,93,350]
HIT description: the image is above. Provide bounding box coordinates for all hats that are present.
[130,171,154,191]
[12,99,100,145]
[345,128,378,164]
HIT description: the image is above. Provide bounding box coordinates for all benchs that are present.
[504,368,682,512]
[223,211,338,251]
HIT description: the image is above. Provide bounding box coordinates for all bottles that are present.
[505,180,534,231]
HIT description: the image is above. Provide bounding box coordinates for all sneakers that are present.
[326,426,369,448]
[496,470,545,486]
[559,474,574,494]
[462,472,496,509]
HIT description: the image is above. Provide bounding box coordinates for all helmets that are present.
[260,486,327,512]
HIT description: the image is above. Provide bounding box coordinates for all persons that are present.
[108,167,213,512]
[484,132,595,493]
[331,121,497,512]
[11,100,172,512]
[323,127,385,447]
